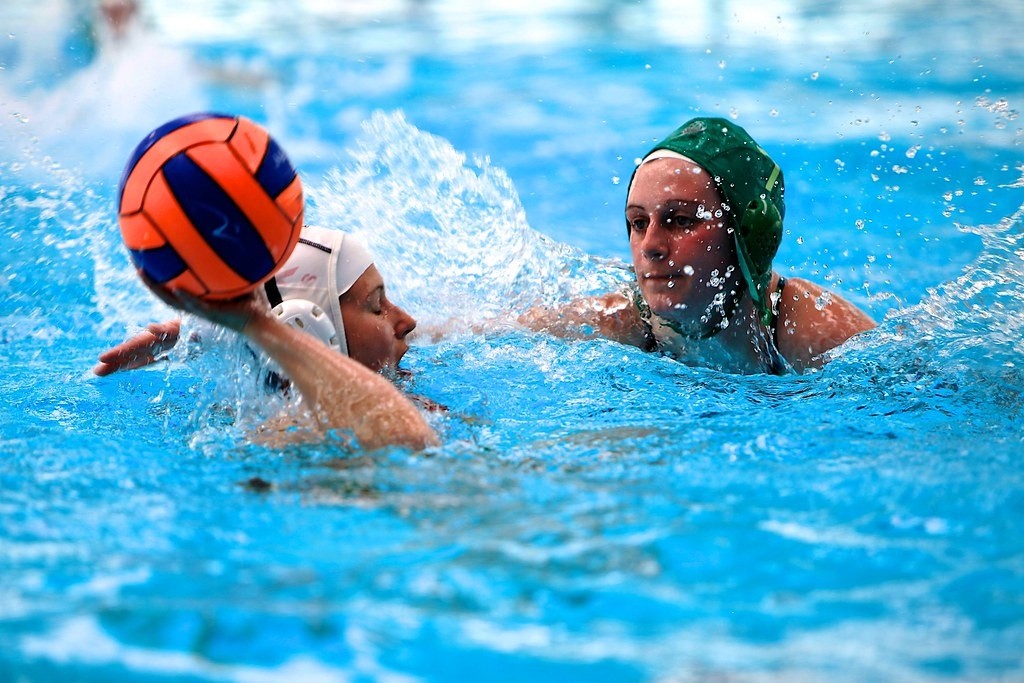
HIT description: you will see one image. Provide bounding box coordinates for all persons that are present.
[514,116,880,376]
[82,117,440,449]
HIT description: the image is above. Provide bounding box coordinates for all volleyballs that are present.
[116,113,303,302]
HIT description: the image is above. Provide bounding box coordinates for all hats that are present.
[624,117,786,376]
[244,226,374,387]
